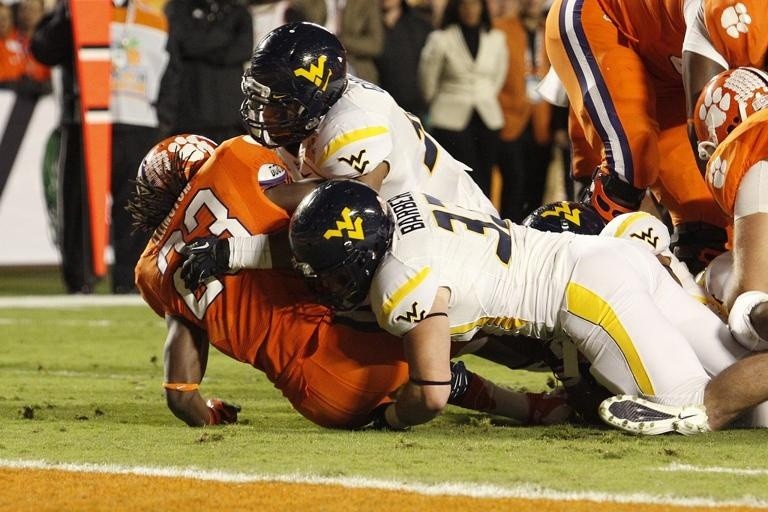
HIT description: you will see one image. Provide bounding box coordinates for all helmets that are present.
[135,133,219,196]
[238,21,348,149]
[288,179,395,312]
[521,200,606,235]
[693,66,768,161]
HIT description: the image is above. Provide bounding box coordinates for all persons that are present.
[0,1,768,434]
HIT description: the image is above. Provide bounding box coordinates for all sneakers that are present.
[597,394,709,436]
[532,385,574,426]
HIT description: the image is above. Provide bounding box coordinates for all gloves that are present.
[358,402,411,432]
[207,399,241,425]
[447,361,472,405]
[176,235,232,291]
